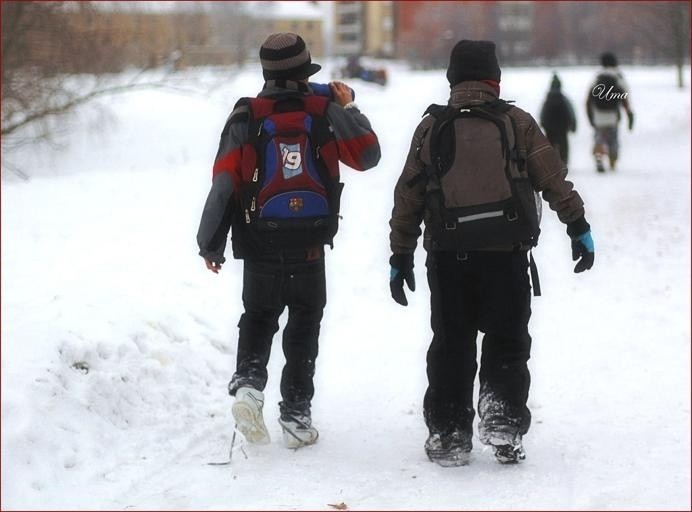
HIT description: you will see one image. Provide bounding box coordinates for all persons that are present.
[197,32,381,450]
[390,40,594,469]
[540,75,576,166]
[586,53,633,173]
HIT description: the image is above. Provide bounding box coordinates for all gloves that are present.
[566,221,595,273]
[628,112,634,129]
[389,256,415,306]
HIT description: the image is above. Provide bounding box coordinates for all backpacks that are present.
[244,94,345,243]
[596,74,619,112]
[431,99,542,248]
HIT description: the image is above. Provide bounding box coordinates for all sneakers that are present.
[232,384,271,444]
[593,156,616,172]
[279,413,317,448]
[493,436,525,463]
[426,437,470,467]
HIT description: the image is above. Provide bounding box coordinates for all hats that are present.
[446,39,500,85]
[600,49,618,67]
[259,32,321,81]
[550,73,560,90]
